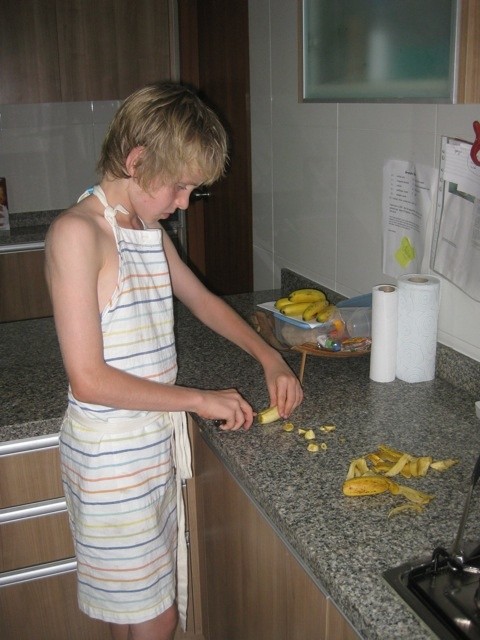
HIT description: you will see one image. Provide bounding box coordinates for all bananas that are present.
[273,289,336,323]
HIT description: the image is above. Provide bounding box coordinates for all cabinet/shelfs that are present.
[0,418,205,640]
[1,246,54,323]
[186,413,366,640]
[297,3,477,105]
[1,1,171,107]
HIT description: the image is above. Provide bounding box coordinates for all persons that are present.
[42,80,304,636]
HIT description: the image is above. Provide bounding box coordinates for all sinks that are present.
[381,544,480,640]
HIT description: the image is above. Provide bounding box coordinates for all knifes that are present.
[212,414,259,427]
